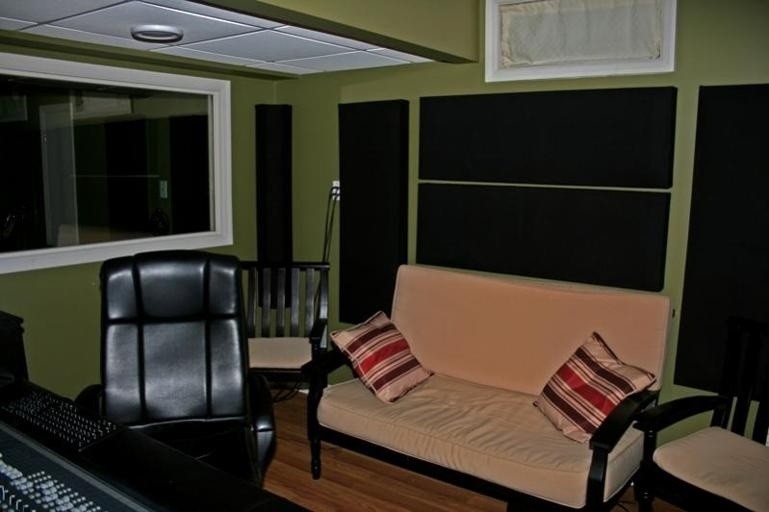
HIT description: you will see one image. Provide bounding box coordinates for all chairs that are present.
[634,315,769,512]
[239,248,329,482]
[77,249,278,482]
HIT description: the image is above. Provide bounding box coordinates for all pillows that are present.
[329,311,434,404]
[532,330,657,443]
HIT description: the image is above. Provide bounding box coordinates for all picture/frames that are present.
[486,2,676,84]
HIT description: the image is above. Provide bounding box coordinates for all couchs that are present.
[307,263,670,509]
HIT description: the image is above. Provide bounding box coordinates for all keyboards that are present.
[1,391,122,452]
